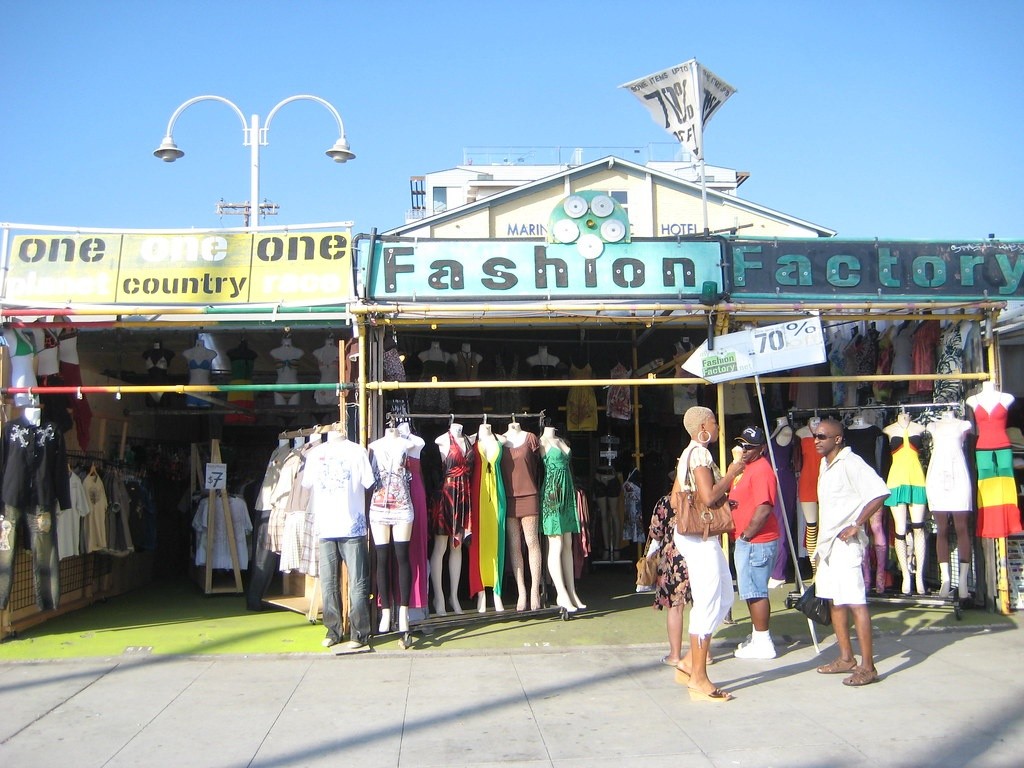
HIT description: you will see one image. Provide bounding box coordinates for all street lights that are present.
[150,92,358,228]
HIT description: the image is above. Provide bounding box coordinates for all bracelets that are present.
[852,520,862,528]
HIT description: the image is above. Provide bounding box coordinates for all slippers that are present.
[706,660,714,665]
[659,656,680,666]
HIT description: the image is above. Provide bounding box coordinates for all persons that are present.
[2,320,646,651]
[647,468,714,668]
[728,425,781,658]
[670,406,744,701]
[673,310,1023,598]
[814,419,892,685]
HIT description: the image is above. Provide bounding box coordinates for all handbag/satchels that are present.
[794,583,832,626]
[637,538,664,592]
[636,531,661,586]
[678,446,734,541]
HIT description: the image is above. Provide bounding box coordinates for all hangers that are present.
[68,448,149,487]
[202,488,237,499]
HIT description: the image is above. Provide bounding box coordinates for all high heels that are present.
[688,680,732,702]
[674,667,691,687]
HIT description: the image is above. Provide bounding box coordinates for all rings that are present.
[844,535,845,537]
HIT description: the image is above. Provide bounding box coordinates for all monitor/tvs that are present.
[198,330,246,374]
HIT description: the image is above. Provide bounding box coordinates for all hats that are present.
[733,426,765,445]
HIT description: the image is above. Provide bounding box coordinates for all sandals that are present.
[817,655,858,673]
[842,666,877,686]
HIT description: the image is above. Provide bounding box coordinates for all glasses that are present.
[736,443,759,450]
[813,433,837,440]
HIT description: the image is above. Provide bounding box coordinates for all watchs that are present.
[740,531,751,541]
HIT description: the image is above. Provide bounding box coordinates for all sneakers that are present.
[734,634,776,659]
